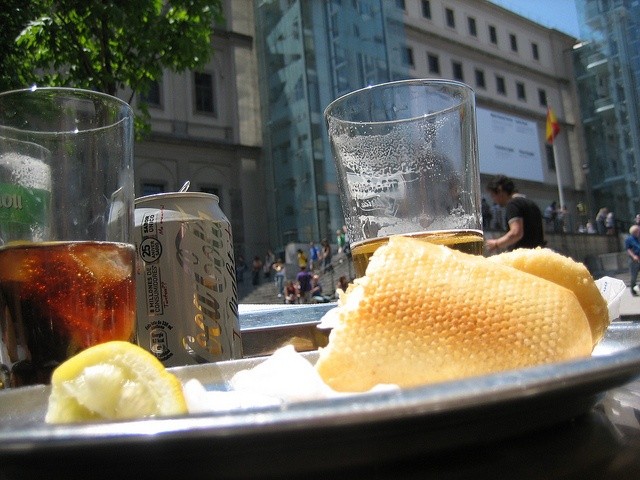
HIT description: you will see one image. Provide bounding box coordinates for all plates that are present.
[0,317,640,453]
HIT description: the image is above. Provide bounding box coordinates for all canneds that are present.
[135,180,242,365]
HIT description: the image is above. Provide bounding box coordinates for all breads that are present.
[47,340,188,424]
[485,245,610,349]
[316,234,593,390]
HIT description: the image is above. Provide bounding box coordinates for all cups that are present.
[0,135,52,246]
[324,78,484,277]
[0,87,137,387]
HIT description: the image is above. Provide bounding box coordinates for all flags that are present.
[545,109,559,144]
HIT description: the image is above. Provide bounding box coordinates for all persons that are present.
[335,230,345,264]
[310,242,322,272]
[543,201,558,233]
[263,249,275,278]
[575,201,588,235]
[624,225,640,297]
[322,239,335,274]
[482,198,492,232]
[335,275,348,299]
[596,206,607,234]
[605,210,617,235]
[272,263,287,298]
[482,174,548,255]
[297,248,308,272]
[559,204,568,232]
[490,202,501,231]
[342,225,352,259]
[252,257,263,285]
[283,280,299,304]
[311,275,324,304]
[296,266,313,304]
[235,254,246,280]
